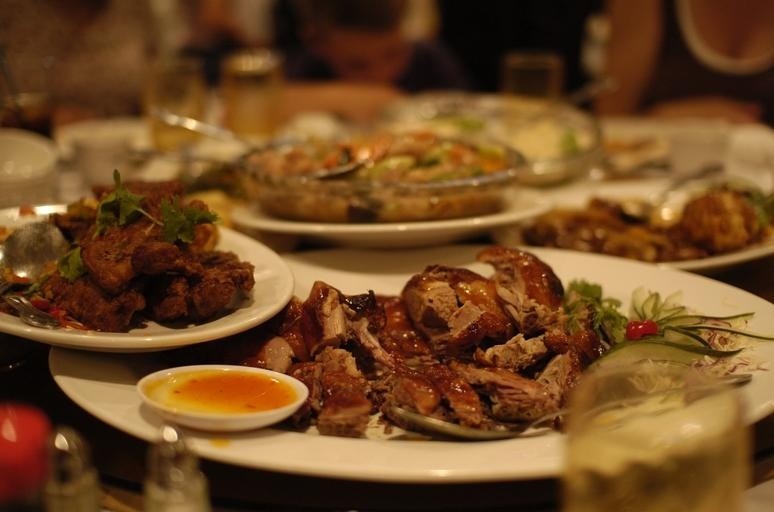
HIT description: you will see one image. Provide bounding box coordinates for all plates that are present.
[1,95,774,210]
[493,182,774,272]
[134,362,303,438]
[0,200,295,351]
[237,196,551,250]
[49,245,774,481]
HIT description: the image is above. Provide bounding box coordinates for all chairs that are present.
[502,54,564,100]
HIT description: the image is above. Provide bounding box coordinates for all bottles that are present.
[145,418,208,510]
[43,430,93,510]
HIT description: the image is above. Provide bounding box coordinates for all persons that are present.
[269,0,506,94]
[592,0,774,130]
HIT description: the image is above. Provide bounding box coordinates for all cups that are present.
[561,346,744,511]
